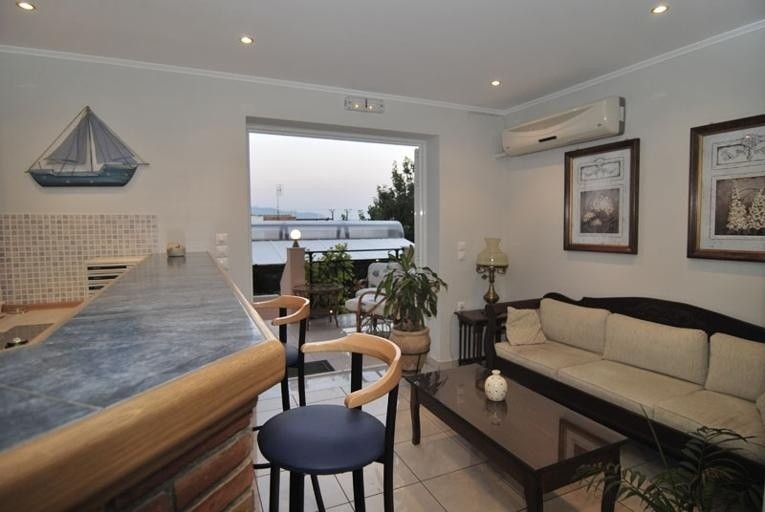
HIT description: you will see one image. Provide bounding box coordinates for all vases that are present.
[485,368,508,401]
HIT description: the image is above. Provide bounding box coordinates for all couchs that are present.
[477,290,765,495]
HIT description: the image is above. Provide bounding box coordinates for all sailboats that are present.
[25,106,151,188]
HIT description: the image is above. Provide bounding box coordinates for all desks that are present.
[453,307,494,366]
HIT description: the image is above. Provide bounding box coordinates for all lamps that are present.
[473,234,511,315]
[289,228,303,248]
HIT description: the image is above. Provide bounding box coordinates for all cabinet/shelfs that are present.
[81,256,142,304]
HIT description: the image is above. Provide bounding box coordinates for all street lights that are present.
[342,207,352,220]
[329,208,337,220]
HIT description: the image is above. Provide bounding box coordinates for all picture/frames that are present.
[560,135,641,255]
[684,111,765,266]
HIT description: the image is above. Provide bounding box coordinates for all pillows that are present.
[538,296,610,356]
[602,312,711,385]
[504,304,548,346]
[704,332,764,401]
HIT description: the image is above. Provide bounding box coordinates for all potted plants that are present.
[372,242,449,381]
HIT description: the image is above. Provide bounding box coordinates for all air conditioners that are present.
[498,91,628,160]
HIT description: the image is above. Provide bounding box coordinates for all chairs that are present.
[255,329,406,512]
[249,291,315,473]
[343,258,407,335]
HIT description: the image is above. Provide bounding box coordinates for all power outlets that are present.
[457,301,465,311]
